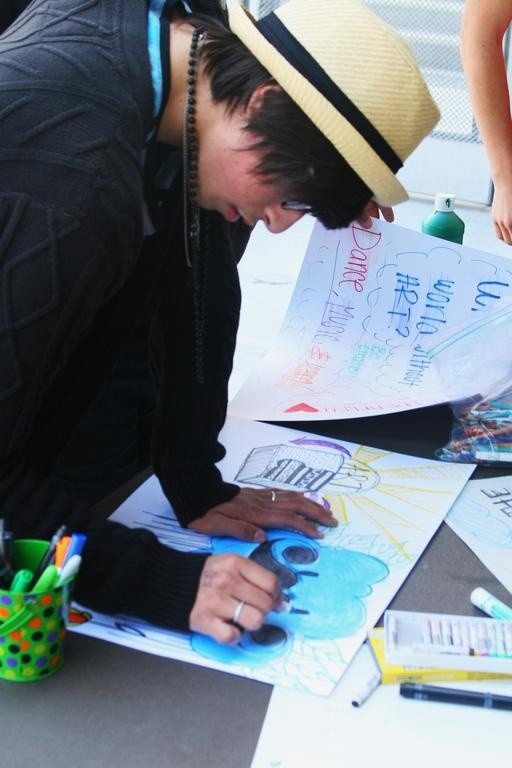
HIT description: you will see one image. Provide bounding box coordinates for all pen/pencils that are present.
[0,518,87,593]
[399,683,512,710]
[352,669,382,706]
[387,615,511,659]
[440,399,512,467]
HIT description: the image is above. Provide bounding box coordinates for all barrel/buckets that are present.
[0,539,77,683]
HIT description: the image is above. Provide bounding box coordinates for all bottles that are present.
[422,192,464,246]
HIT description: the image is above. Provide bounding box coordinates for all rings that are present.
[271,490,276,502]
[232,602,243,624]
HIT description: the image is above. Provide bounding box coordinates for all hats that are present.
[223,0,444,209]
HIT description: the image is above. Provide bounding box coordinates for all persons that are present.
[460,0,512,246]
[0,1,443,642]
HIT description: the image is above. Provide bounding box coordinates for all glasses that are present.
[280,177,318,213]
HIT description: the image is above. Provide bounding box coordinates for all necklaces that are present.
[180,21,212,385]
